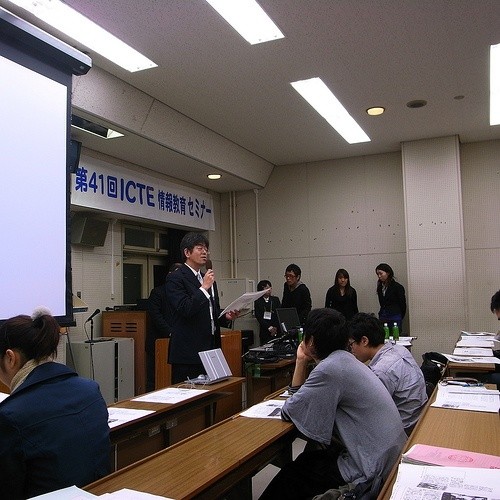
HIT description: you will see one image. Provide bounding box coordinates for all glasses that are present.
[284,274,298,279]
[348,339,357,349]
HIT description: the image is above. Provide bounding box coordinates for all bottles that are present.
[393,323,399,341]
[299,328,304,342]
[384,323,389,339]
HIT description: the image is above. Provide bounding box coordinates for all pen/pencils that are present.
[447,381,467,385]
[472,358,483,359]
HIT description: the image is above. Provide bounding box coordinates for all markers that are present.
[464,383,483,386]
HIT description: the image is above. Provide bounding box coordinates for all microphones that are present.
[206,260,215,297]
[86,309,100,322]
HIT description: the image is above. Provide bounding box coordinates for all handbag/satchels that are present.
[311,474,384,500]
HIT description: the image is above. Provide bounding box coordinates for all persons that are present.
[348,313,428,434]
[282,264,312,324]
[467,289,500,387]
[149,232,241,385]
[0,307,112,500]
[258,308,408,500]
[254,280,281,344]
[325,269,359,317]
[376,263,407,336]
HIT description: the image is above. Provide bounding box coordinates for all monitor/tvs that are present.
[276,307,300,332]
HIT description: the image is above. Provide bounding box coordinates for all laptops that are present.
[184,347,233,385]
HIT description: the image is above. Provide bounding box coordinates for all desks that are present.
[81,310,500,500]
